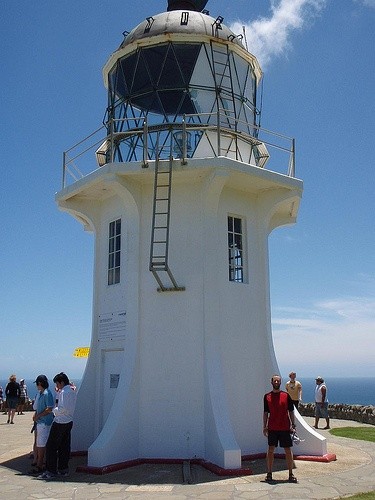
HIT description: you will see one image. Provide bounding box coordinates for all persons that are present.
[263,375,297,483]
[0,386,37,412]
[5,374,21,424]
[285,372,302,410]
[37,371,77,480]
[17,379,27,415]
[28,375,55,474]
[313,377,330,429]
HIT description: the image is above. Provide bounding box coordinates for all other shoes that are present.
[313,426,318,428]
[57,472,68,477]
[7,420,9,423]
[29,466,43,474]
[324,426,330,429]
[36,473,56,479]
[32,463,37,466]
[266,475,272,480]
[10,422,15,424]
[289,474,298,482]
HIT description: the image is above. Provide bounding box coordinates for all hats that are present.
[315,377,324,383]
[33,375,48,383]
[20,379,24,382]
